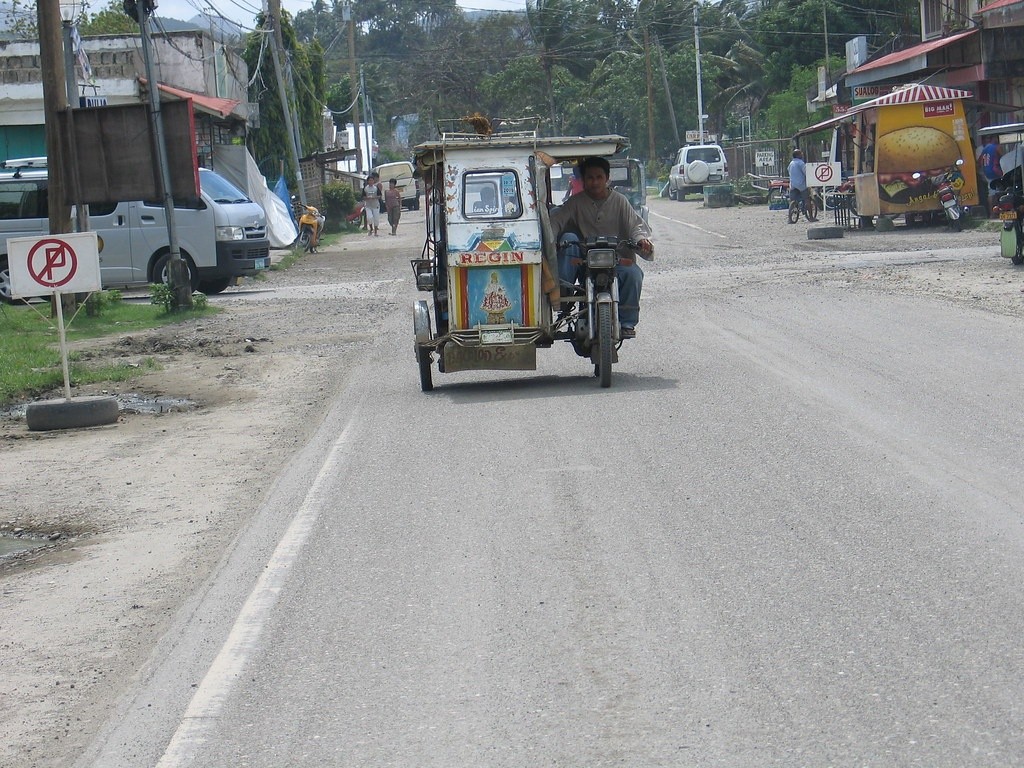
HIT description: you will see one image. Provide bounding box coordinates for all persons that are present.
[971,134,1006,218]
[788,149,819,223]
[362,172,382,236]
[553,158,653,339]
[385,178,401,235]
[562,166,583,202]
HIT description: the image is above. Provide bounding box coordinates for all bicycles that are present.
[788,186,819,224]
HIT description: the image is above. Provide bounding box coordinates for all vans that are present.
[371,161,421,213]
[0,157,272,305]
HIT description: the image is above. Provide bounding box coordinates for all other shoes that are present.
[810,218,818,221]
[389,232,396,235]
[375,228,377,235]
[368,229,373,236]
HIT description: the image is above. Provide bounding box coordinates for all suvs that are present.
[668,143,727,202]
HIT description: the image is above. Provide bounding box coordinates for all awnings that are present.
[974,0,1024,14]
[791,110,863,137]
[141,77,240,120]
[844,29,979,75]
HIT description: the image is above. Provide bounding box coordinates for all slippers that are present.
[560,287,571,311]
[622,327,636,338]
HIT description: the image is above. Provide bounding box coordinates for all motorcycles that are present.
[990,178,1024,266]
[912,159,969,232]
[766,177,860,216]
[297,201,326,253]
[409,114,655,391]
